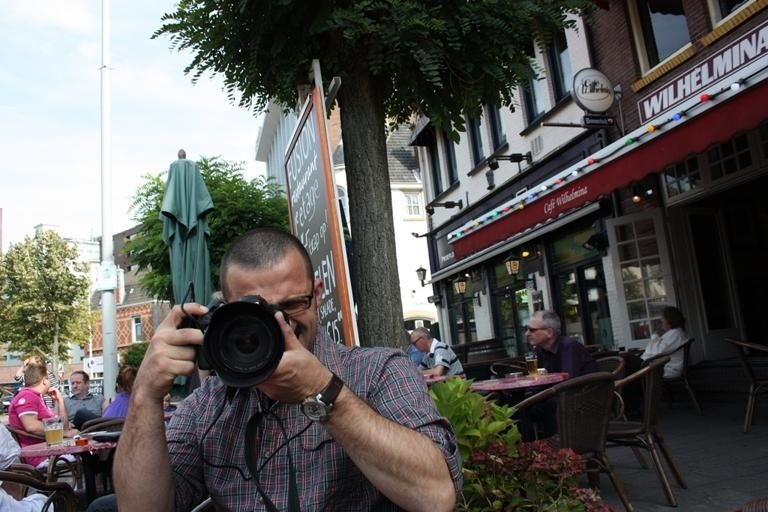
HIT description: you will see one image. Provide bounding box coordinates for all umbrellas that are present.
[158,149,215,387]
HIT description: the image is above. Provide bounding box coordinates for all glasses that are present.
[277,278,314,318]
[524,324,549,333]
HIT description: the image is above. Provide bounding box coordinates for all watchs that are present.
[301,373,344,424]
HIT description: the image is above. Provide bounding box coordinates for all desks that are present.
[424,374,464,386]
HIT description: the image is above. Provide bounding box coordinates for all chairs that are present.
[607,354,688,509]
[487,336,707,472]
[510,370,636,512]
[78,417,124,502]
[0,463,77,512]
[721,337,768,434]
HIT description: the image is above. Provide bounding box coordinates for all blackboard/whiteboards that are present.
[283,86,357,348]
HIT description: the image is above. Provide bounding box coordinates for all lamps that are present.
[453,273,482,307]
[416,266,432,287]
[426,200,462,214]
[505,251,544,277]
[488,152,532,169]
[427,295,441,303]
[582,233,601,251]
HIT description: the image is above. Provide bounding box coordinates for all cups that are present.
[525,352,538,375]
[42,415,64,446]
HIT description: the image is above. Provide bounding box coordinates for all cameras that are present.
[182,294,286,388]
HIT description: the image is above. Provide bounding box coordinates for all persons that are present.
[1,354,180,512]
[111,226,464,511]
[413,327,468,388]
[521,309,597,442]
[405,332,425,367]
[621,306,689,426]
